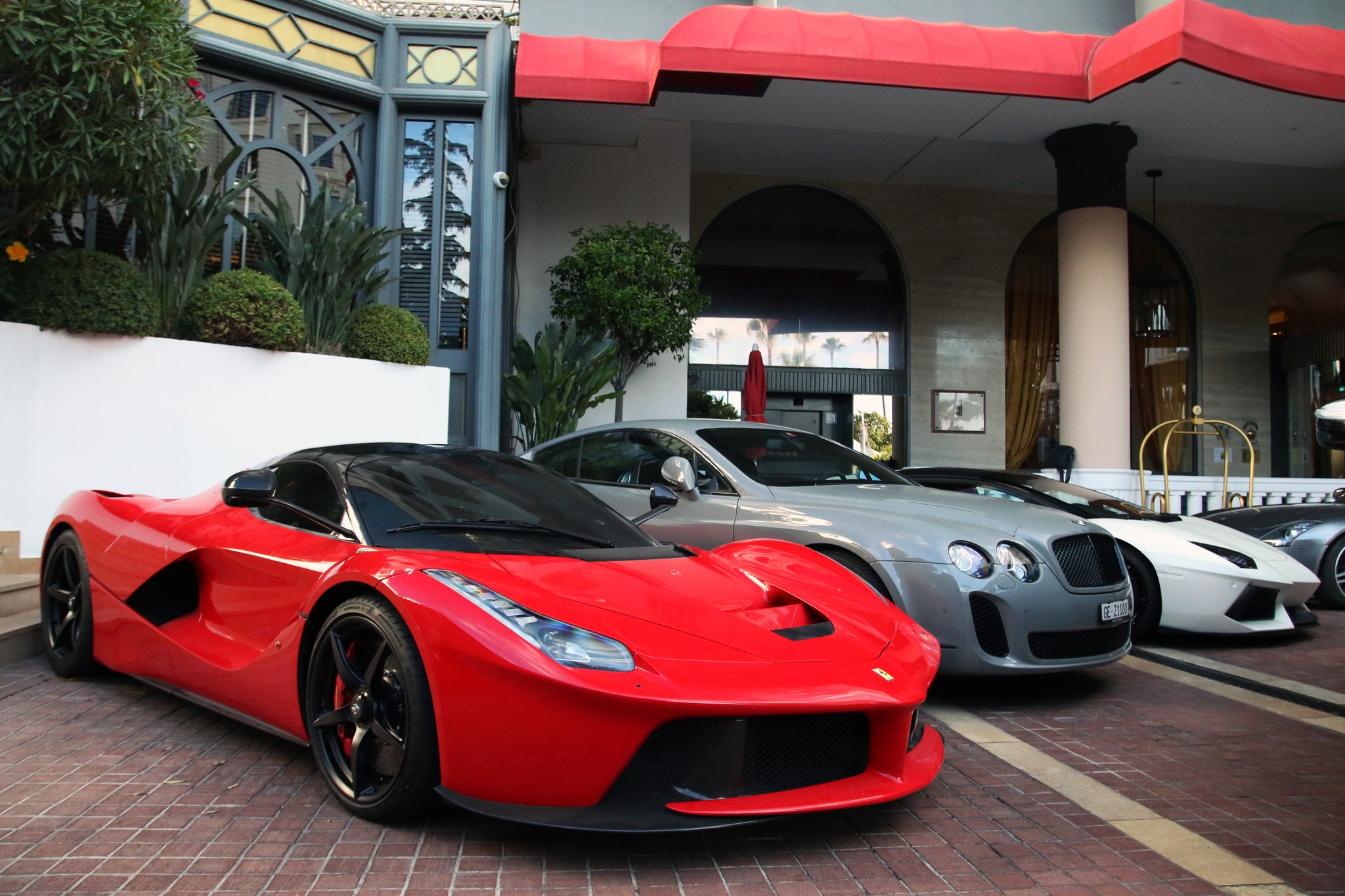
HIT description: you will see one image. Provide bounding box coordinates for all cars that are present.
[480,418,1135,679]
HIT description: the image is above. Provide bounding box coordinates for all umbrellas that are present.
[742,344,768,464]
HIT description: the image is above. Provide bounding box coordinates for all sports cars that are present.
[892,464,1321,642]
[37,441,948,833]
[1192,488,1345,614]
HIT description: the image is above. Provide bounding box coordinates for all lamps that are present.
[1130,171,1183,340]
